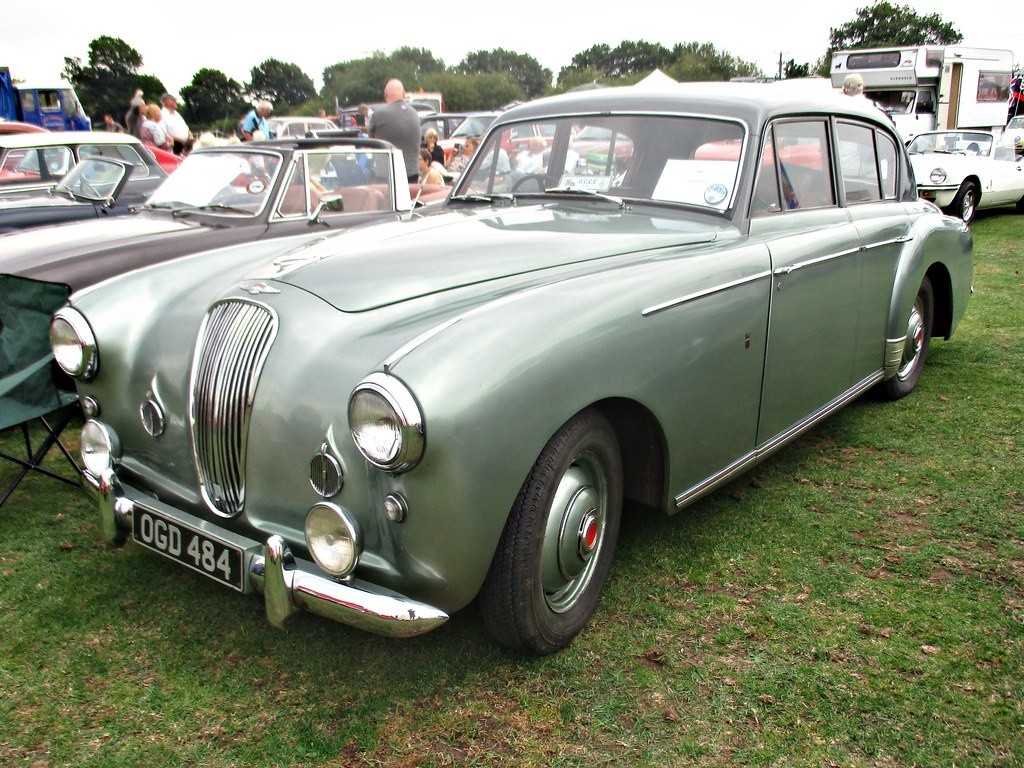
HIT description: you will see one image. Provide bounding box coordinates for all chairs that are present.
[329,186,385,214]
[989,147,1006,159]
[280,185,323,216]
[966,142,980,155]
[753,165,822,220]
[608,145,675,201]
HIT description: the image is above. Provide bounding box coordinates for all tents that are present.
[634,69,678,85]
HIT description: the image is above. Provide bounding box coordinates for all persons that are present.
[838,75,882,180]
[126,89,193,155]
[368,79,421,183]
[319,104,373,129]
[509,136,578,182]
[104,115,124,133]
[238,101,274,170]
[419,128,511,187]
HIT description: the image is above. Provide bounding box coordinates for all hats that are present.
[844,73,863,94]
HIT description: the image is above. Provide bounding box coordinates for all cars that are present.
[880,130,1024,226]
[336,93,464,147]
[541,126,634,176]
[0,154,154,238]
[440,114,580,173]
[0,135,170,200]
[48,79,971,658]
[0,129,476,294]
[1001,115,1023,155]
[0,119,252,187]
[693,137,822,173]
[268,118,339,139]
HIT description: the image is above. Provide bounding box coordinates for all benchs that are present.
[360,183,451,203]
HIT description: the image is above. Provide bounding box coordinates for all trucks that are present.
[830,45,1014,148]
[0,65,90,131]
